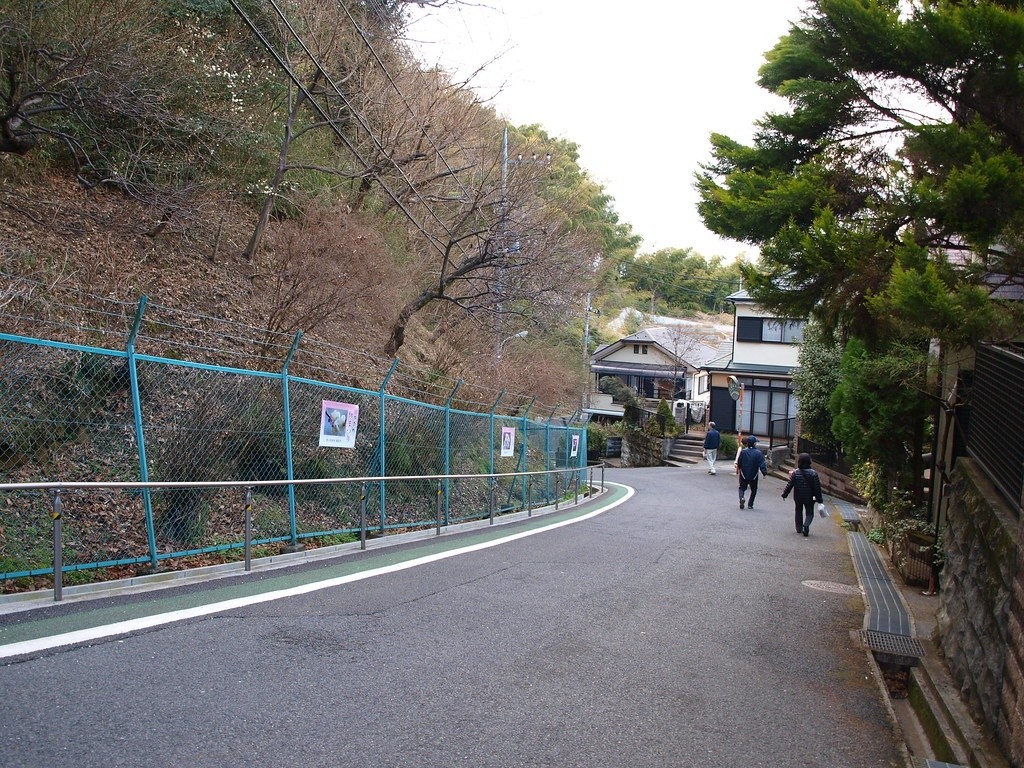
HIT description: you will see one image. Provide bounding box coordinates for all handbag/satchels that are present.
[817,504,830,519]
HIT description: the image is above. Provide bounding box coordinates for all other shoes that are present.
[740,498,745,509]
[708,471,715,475]
[803,526,809,536]
[748,506,753,509]
[797,530,804,533]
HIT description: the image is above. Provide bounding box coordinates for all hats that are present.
[742,438,749,443]
[798,453,810,466]
[747,435,760,442]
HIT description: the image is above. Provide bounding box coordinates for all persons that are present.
[782,452,823,537]
[734,437,749,474]
[703,422,720,475]
[685,403,691,433]
[738,435,767,510]
